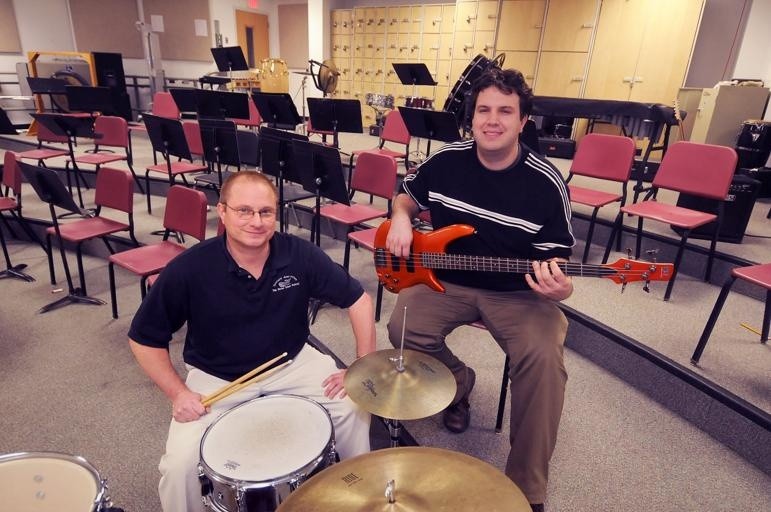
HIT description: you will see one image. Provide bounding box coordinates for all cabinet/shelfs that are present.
[329,0,707,154]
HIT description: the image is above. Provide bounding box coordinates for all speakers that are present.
[91,51,133,120]
[726,119,771,167]
[670,174,762,243]
[735,165,771,199]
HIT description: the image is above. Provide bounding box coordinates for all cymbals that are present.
[319,60,338,93]
[343,348,458,420]
[276,446,533,512]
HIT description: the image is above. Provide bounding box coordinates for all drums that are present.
[406,97,434,111]
[365,94,394,108]
[1,452,109,512]
[197,393,339,511]
[259,58,289,93]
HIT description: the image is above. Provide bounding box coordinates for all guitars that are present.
[374,220,674,293]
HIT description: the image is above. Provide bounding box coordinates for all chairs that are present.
[310,152,397,272]
[349,111,411,205]
[1,87,353,319]
[342,167,431,321]
[601,142,737,300]
[565,132,636,264]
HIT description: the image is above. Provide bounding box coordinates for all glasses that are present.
[222,202,277,221]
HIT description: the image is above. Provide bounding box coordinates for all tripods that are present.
[308,182,345,326]
[47,90,76,144]
[151,150,186,243]
[39,196,107,313]
[84,144,115,154]
[55,131,97,218]
[0,221,36,282]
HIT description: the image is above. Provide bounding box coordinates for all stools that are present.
[691,262,770,364]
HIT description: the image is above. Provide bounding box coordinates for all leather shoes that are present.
[442,367,476,434]
[530,503,544,512]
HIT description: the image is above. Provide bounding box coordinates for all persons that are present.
[127,170,376,512]
[386,68,577,512]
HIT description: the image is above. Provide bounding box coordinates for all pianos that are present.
[199,68,260,90]
